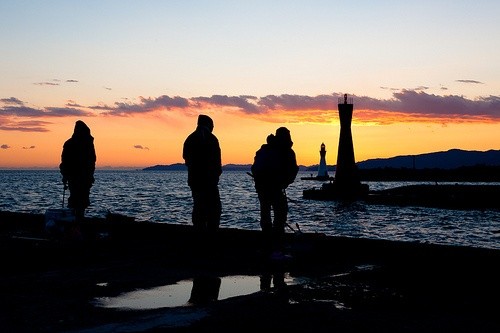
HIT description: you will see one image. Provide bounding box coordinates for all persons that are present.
[252,126,299,231]
[59,118,96,211]
[182,115,223,227]
